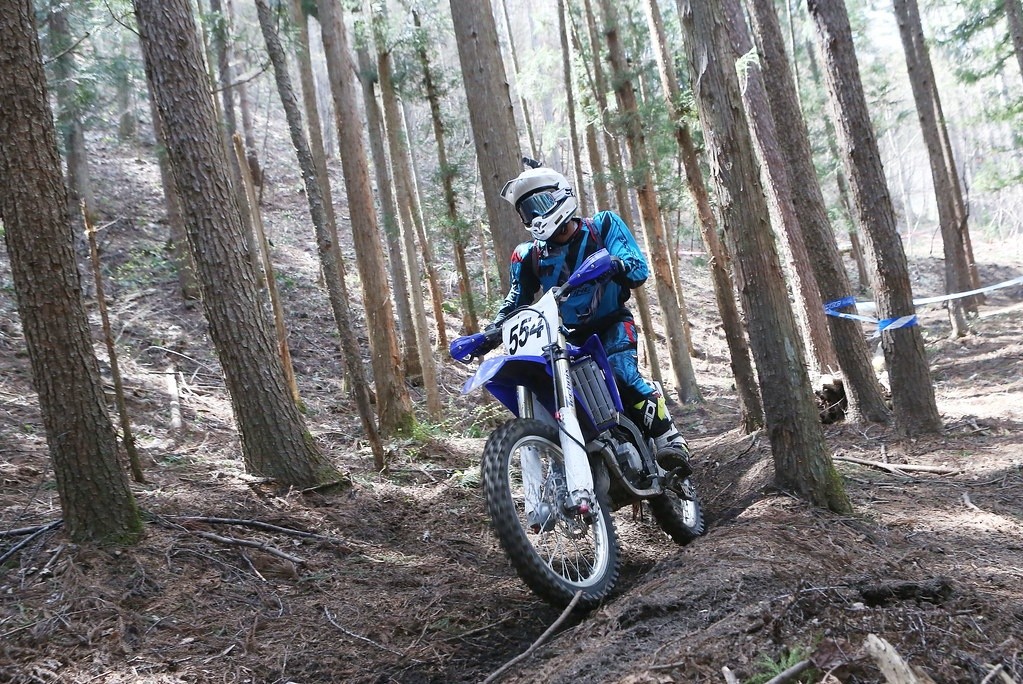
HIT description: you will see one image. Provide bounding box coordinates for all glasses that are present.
[517,191,557,224]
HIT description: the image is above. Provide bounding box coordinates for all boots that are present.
[633,390,694,477]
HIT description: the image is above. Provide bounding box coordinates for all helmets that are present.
[500,167,577,241]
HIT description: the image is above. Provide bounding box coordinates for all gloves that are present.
[594,255,620,284]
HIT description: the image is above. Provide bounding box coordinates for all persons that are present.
[477,167,693,523]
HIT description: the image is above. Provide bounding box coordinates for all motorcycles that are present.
[450,248,704,613]
